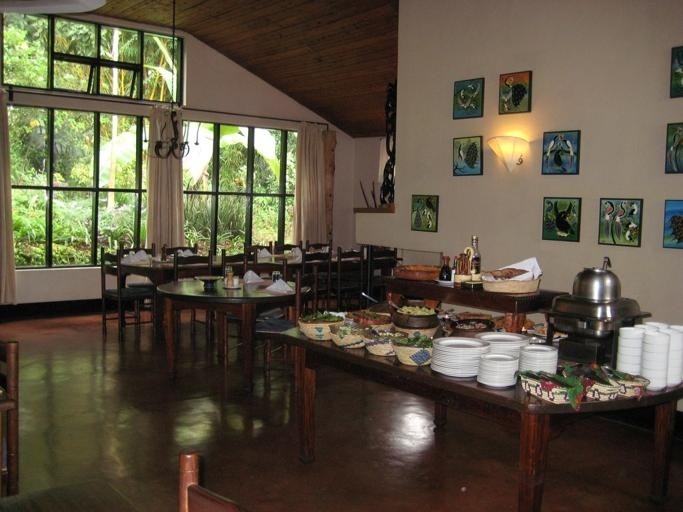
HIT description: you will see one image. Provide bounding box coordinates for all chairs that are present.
[241,242,273,260]
[222,247,259,345]
[337,245,364,310]
[254,265,319,404]
[367,245,399,306]
[170,251,214,349]
[118,241,157,328]
[274,241,304,258]
[305,238,334,256]
[1,341,20,456]
[161,245,198,262]
[100,247,161,343]
[300,248,336,306]
[178,452,251,512]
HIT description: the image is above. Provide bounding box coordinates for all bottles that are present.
[439,256,451,280]
[456,253,470,274]
[469,236,481,274]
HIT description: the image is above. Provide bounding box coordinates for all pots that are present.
[390,307,440,330]
[444,319,495,335]
[537,293,652,339]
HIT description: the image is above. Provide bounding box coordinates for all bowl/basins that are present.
[633,320,670,332]
[361,335,395,356]
[391,344,432,366]
[616,325,643,376]
[394,327,439,340]
[640,330,671,391]
[298,320,344,340]
[669,324,682,389]
[329,328,365,348]
[369,312,394,332]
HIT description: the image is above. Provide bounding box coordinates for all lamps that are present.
[486,137,528,174]
[140,1,202,160]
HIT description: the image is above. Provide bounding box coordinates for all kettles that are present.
[571,257,622,304]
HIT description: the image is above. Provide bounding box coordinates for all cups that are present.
[272,271,281,283]
[224,265,232,278]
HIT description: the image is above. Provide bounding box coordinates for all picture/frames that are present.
[452,135,483,176]
[662,200,683,250]
[541,130,580,175]
[499,70,532,115]
[665,122,683,173]
[669,46,683,100]
[542,197,580,242]
[411,195,439,233]
[453,77,486,121]
[598,197,644,248]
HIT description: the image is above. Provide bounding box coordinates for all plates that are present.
[518,344,558,375]
[433,280,454,287]
[430,336,489,378]
[223,286,241,290]
[473,331,529,356]
[477,352,519,389]
